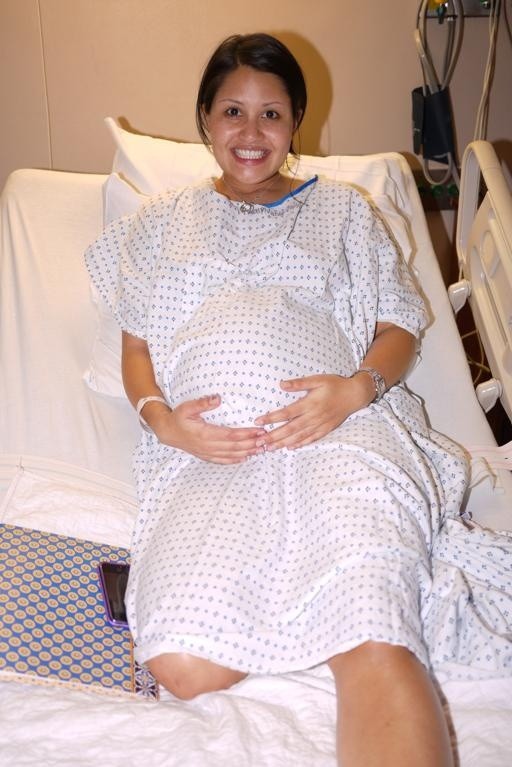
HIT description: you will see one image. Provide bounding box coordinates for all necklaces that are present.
[220,173,282,214]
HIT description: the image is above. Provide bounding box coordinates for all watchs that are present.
[349,365,387,404]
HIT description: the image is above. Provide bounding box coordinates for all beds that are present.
[0,152,512,767]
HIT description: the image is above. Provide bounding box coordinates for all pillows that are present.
[85,115,423,402]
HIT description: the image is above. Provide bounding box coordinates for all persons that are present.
[83,33,512,767]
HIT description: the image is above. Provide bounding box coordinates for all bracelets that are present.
[136,395,173,434]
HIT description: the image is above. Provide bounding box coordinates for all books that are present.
[0,523,159,704]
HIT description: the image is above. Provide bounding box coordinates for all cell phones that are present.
[98,560,131,629]
